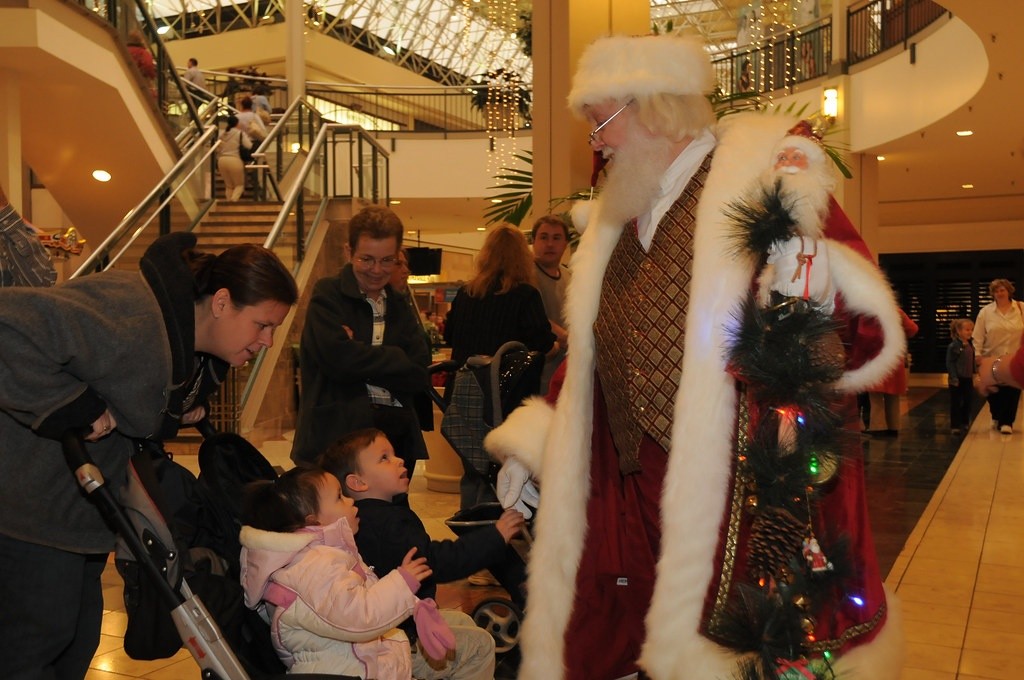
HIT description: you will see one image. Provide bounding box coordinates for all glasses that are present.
[353,251,399,267]
[589,98,635,147]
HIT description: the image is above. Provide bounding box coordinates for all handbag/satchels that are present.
[239,132,251,162]
[255,109,271,126]
[250,114,266,141]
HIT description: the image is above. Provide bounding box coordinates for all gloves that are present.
[495,455,541,521]
[764,234,830,302]
[414,598,456,660]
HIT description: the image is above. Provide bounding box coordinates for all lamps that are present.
[379,46,396,59]
[157,26,174,40]
[822,87,837,118]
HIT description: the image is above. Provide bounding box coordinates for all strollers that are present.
[58,412,367,680]
[422,337,535,664]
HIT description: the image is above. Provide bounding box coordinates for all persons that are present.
[771,119,833,236]
[126,29,155,84]
[483,37,906,680]
[0,228,300,680]
[971,280,1024,434]
[442,213,569,509]
[858,307,918,439]
[184,58,204,109]
[805,539,827,572]
[947,318,976,435]
[234,428,524,680]
[290,203,434,486]
[223,79,272,163]
[217,116,252,201]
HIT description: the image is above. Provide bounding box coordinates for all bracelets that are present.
[992,355,1005,385]
[94,408,111,436]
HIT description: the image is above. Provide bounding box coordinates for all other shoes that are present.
[991,420,998,428]
[951,428,961,436]
[1001,425,1013,433]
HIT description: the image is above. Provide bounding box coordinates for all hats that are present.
[565,34,716,120]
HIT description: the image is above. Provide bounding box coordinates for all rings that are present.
[976,373,982,385]
